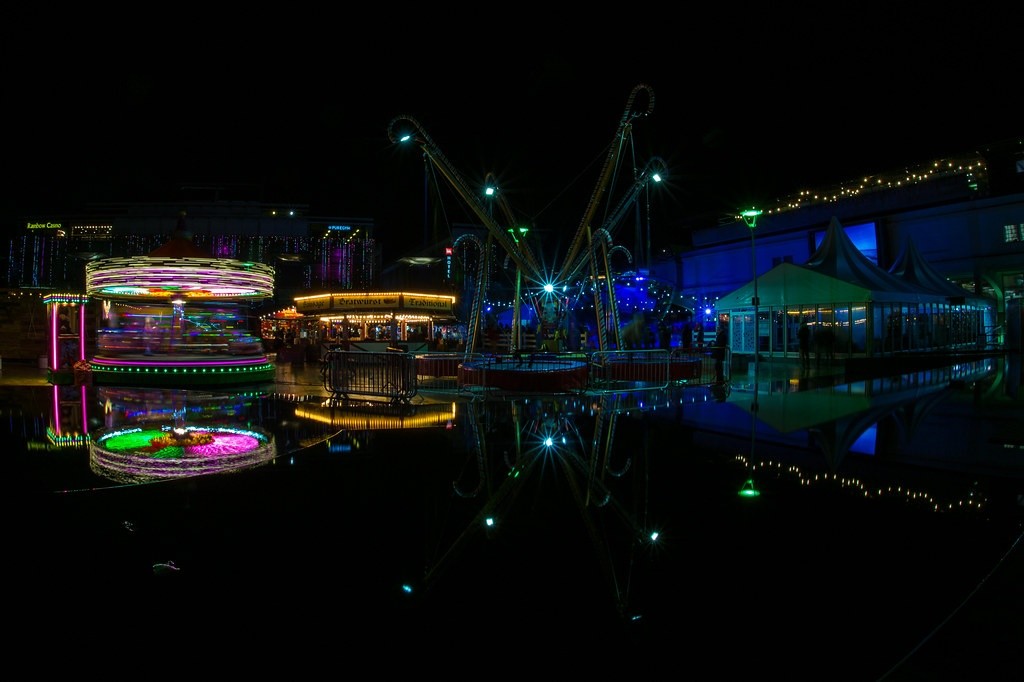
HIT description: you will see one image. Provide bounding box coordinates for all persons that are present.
[796,322,812,369]
[814,324,834,368]
[58,312,76,349]
[535,321,704,353]
[487,326,498,349]
[273,323,445,352]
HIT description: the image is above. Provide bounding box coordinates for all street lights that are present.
[508,227,530,354]
[740,206,766,372]
